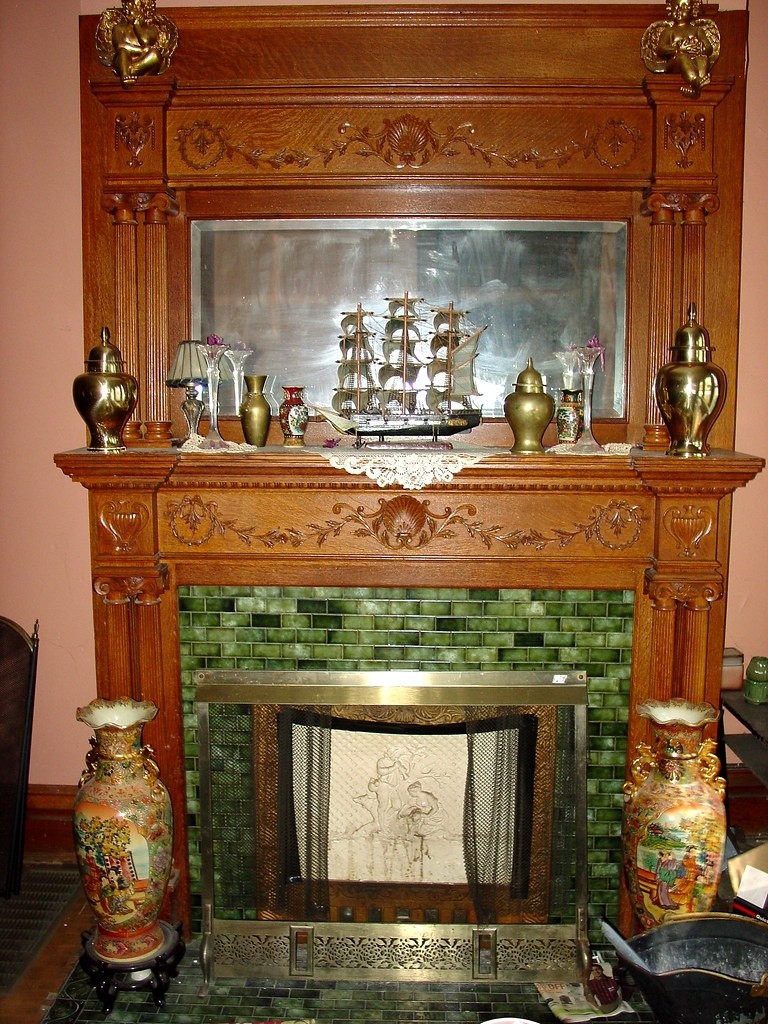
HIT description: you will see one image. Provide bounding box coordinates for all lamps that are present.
[164,338,221,449]
[219,355,234,383]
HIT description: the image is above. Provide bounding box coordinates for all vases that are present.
[239,375,272,448]
[72,695,178,967]
[619,696,728,932]
[278,385,308,448]
[555,389,584,445]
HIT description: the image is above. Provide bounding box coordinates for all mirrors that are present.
[190,220,629,422]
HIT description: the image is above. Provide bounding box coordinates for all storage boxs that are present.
[728,843,767,925]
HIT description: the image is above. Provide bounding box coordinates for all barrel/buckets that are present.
[615,917,768,1024]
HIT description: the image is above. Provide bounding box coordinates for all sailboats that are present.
[304,290,489,436]
